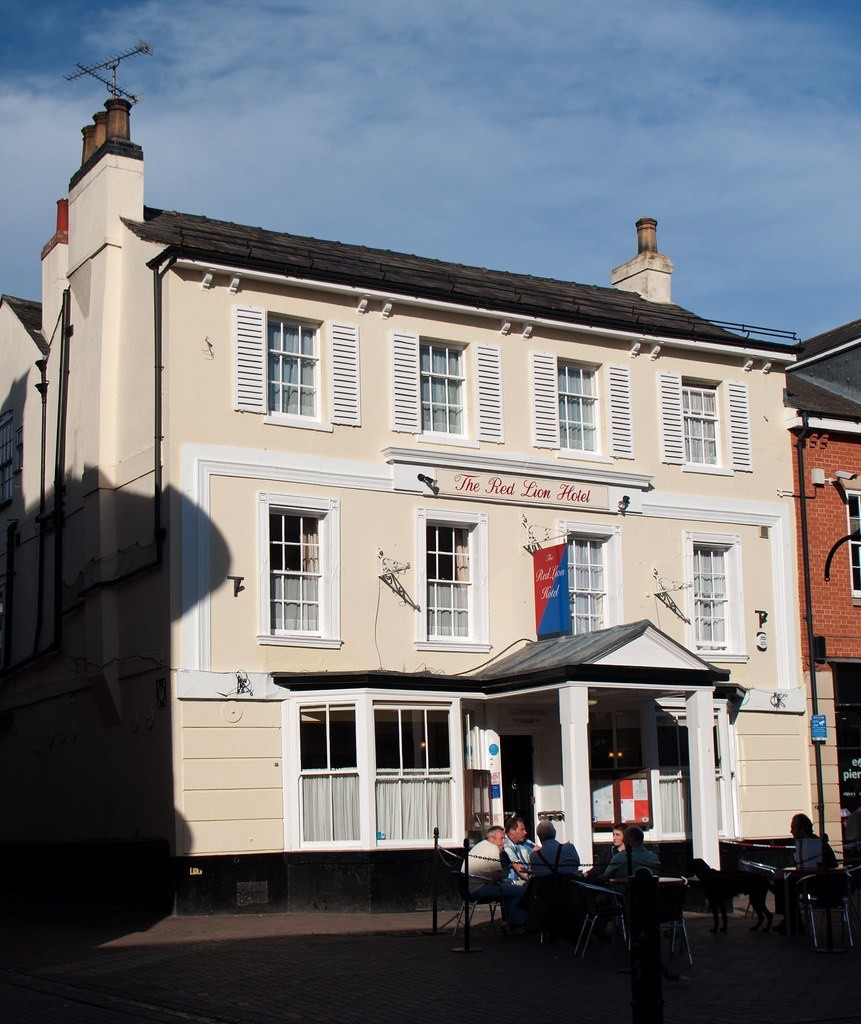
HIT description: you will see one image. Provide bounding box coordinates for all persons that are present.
[769,814,838,936]
[530,821,578,885]
[504,817,540,881]
[460,825,527,932]
[601,826,660,894]
[594,823,628,929]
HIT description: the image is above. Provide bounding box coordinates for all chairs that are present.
[450,870,499,937]
[574,885,627,957]
[798,873,855,948]
[657,882,694,967]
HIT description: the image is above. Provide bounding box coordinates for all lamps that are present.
[417,473,438,490]
[618,496,631,511]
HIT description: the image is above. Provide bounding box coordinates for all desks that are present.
[782,866,847,939]
[608,875,686,956]
